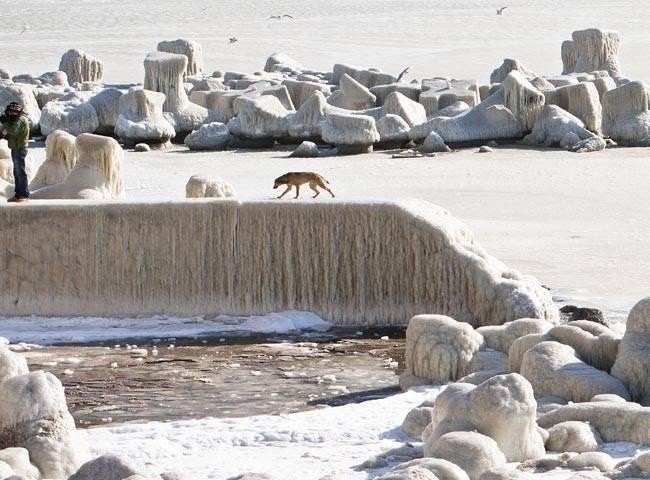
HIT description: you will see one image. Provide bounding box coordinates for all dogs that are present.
[273,172,335,199]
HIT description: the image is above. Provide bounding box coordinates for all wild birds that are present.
[229,37,237,44]
[396,66,410,83]
[266,14,294,20]
[497,7,507,15]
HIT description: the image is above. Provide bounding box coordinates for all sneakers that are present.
[7,195,30,203]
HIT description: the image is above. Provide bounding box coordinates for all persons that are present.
[0,100,31,204]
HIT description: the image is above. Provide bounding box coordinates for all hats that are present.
[8,103,23,112]
